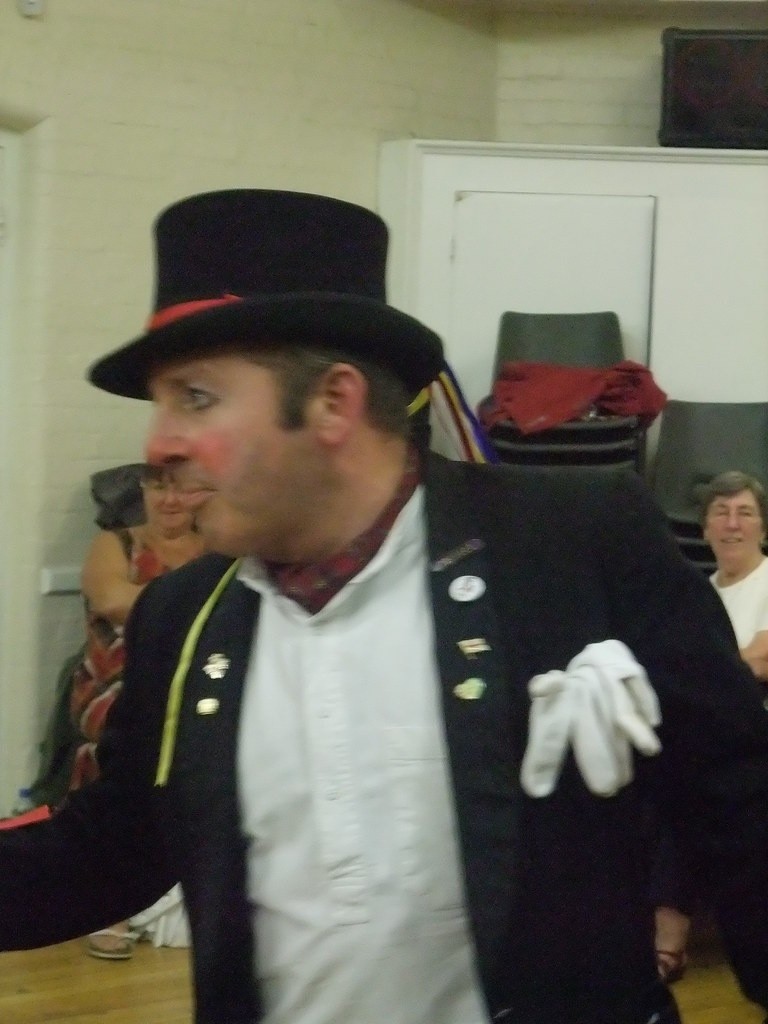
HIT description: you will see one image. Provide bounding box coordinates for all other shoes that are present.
[655,948,684,986]
[82,919,134,958]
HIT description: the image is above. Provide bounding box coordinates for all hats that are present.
[86,189,444,408]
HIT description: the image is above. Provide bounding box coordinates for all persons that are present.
[60,463,768,984]
[0,188,768,1024]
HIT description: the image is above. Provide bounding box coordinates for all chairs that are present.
[476,310,647,477]
[651,399,768,577]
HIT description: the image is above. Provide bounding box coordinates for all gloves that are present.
[518,638,664,798]
